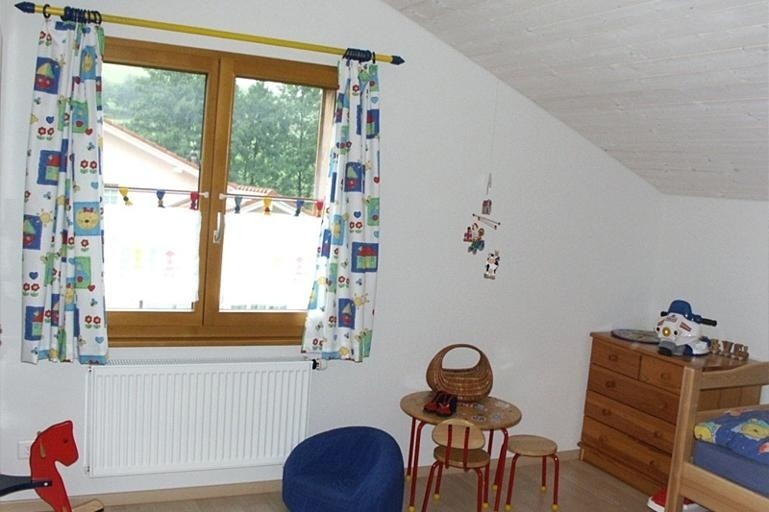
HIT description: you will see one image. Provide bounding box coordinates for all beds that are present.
[663,360,769,512]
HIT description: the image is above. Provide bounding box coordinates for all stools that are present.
[494,434,560,512]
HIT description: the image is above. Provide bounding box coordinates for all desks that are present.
[400,391,522,512]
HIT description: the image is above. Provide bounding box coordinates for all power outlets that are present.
[17,441,31,458]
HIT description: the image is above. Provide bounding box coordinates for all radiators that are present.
[84,358,313,479]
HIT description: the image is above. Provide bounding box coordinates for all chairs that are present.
[420,418,491,512]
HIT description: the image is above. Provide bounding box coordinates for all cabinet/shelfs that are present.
[577,331,762,497]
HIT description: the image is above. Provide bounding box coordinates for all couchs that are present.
[282,425,405,512]
